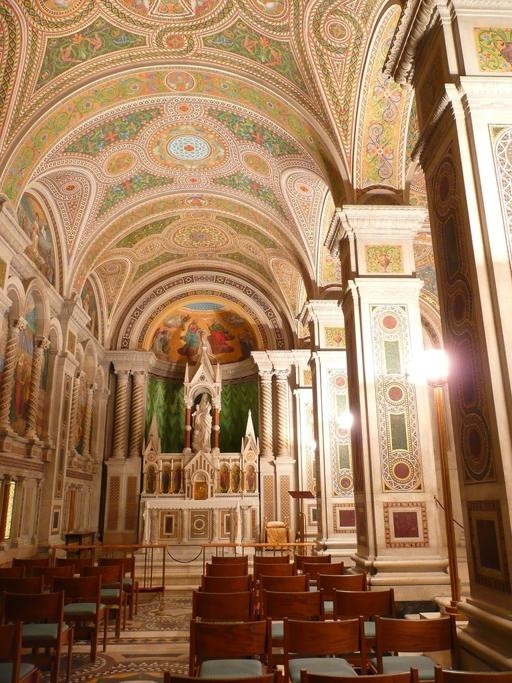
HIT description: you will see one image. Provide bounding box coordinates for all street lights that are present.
[421,346,472,621]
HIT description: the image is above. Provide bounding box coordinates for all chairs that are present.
[0,554,137,682]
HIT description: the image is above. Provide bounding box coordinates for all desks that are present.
[64,531,96,558]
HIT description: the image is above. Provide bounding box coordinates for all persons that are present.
[186,394,216,452]
